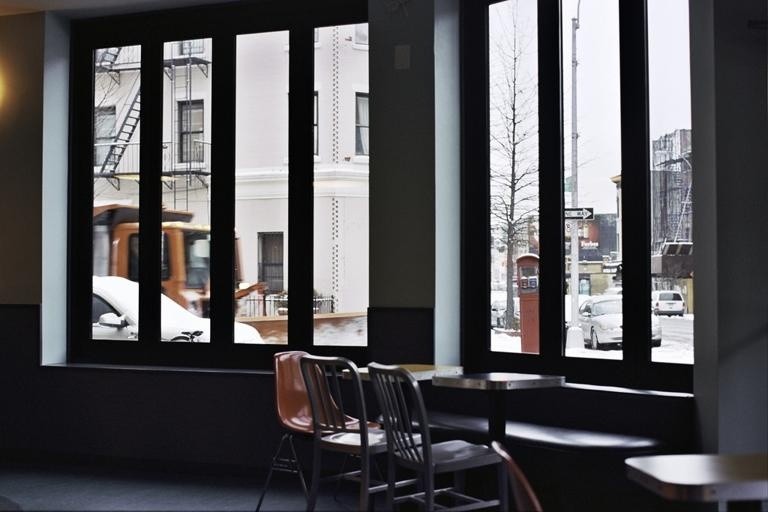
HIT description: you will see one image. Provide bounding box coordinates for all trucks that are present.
[92,201,269,323]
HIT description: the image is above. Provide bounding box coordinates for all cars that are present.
[89,270,264,344]
[489,278,688,350]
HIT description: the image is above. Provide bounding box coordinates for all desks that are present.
[624,452,768,512]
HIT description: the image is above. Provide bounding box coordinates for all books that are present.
[431,369,565,390]
[342,362,463,383]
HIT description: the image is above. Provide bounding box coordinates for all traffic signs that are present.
[563,206,594,221]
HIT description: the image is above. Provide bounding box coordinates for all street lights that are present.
[653,148,691,241]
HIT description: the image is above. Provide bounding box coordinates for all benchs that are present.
[376,411,663,510]
